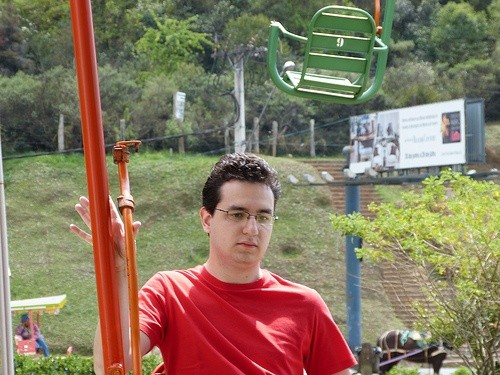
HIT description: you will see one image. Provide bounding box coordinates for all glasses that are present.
[214,206,278,227]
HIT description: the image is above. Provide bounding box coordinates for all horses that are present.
[377,329,456,375]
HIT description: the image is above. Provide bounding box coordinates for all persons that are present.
[351,119,400,173]
[15,314,49,357]
[69,152,359,375]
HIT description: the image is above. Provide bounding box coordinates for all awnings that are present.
[10,294,67,313]
